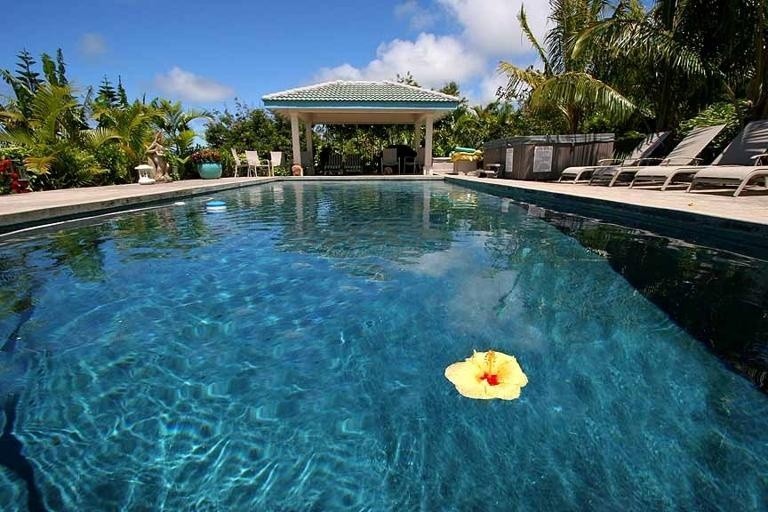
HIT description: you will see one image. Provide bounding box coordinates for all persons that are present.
[146,133,173,183]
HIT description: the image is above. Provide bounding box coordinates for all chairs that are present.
[688,154,768,196]
[230,147,426,173]
[587,122,725,187]
[557,127,671,185]
[628,120,768,191]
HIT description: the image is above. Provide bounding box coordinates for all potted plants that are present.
[194,150,224,179]
